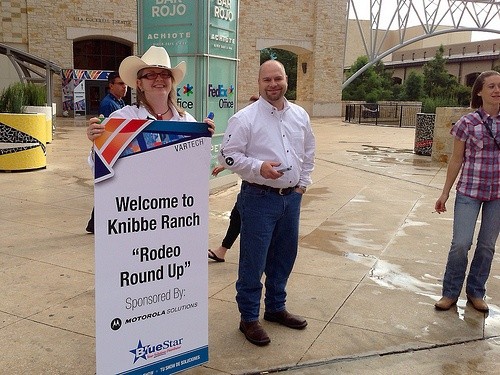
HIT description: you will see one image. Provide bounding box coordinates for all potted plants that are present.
[0,84,46,171]
[21,81,53,142]
[414,99,440,155]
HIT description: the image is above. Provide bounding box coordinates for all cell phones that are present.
[277,167,292,173]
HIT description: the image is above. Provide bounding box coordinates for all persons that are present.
[435,70,500,312]
[87,46,216,167]
[86,72,127,232]
[208,97,259,261]
[218,60,315,347]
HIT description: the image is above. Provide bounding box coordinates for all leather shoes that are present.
[264,309,308,329]
[435,295,457,310]
[467,294,489,312]
[240,320,271,345]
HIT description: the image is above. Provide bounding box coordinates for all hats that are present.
[118,45,186,90]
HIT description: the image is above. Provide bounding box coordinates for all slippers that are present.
[208,249,225,262]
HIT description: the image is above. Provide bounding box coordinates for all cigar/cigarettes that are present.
[432,210,440,213]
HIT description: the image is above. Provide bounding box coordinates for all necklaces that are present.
[150,109,170,120]
[90,130,94,135]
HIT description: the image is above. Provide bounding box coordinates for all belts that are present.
[242,179,298,195]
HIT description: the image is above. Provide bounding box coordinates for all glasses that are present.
[114,82,125,85]
[139,70,172,80]
